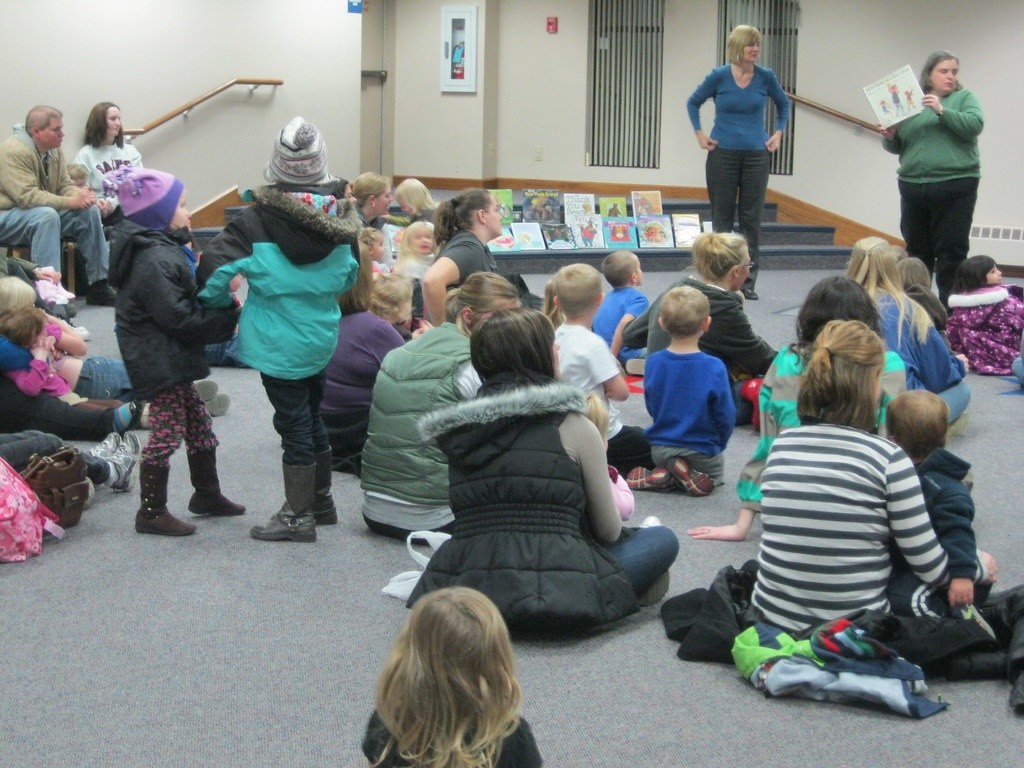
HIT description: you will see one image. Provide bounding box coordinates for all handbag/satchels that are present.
[19,444,91,529]
[382,528,454,603]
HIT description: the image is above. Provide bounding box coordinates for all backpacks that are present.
[1,459,60,562]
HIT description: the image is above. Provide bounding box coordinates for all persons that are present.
[686,276,973,542]
[896,257,949,337]
[1011,327,1024,392]
[626,285,736,497]
[945,256,1024,376]
[319,172,656,545]
[751,320,998,654]
[407,308,680,638]
[66,101,145,252]
[876,52,984,318]
[687,25,790,300]
[847,236,970,426]
[194,116,360,543]
[363,587,543,768]
[891,245,909,264]
[885,390,977,622]
[622,231,780,426]
[0,105,118,317]
[0,254,229,563]
[111,168,246,537]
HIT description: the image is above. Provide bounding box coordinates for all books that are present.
[863,64,925,129]
[465,186,716,255]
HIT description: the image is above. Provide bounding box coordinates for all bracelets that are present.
[936,107,944,116]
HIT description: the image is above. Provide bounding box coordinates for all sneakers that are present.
[87,433,121,464]
[105,430,139,492]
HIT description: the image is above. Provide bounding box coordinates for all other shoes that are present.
[667,456,713,497]
[741,283,759,301]
[626,358,646,376]
[87,281,119,309]
[193,380,232,416]
[626,468,675,492]
[637,568,670,608]
[124,398,146,432]
[947,410,967,436]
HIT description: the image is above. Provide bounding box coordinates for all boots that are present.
[187,449,246,515]
[134,460,197,535]
[249,461,317,543]
[314,446,340,526]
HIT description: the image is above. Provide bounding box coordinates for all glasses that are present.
[742,260,755,269]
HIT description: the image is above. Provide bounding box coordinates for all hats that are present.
[117,169,182,232]
[262,117,340,187]
